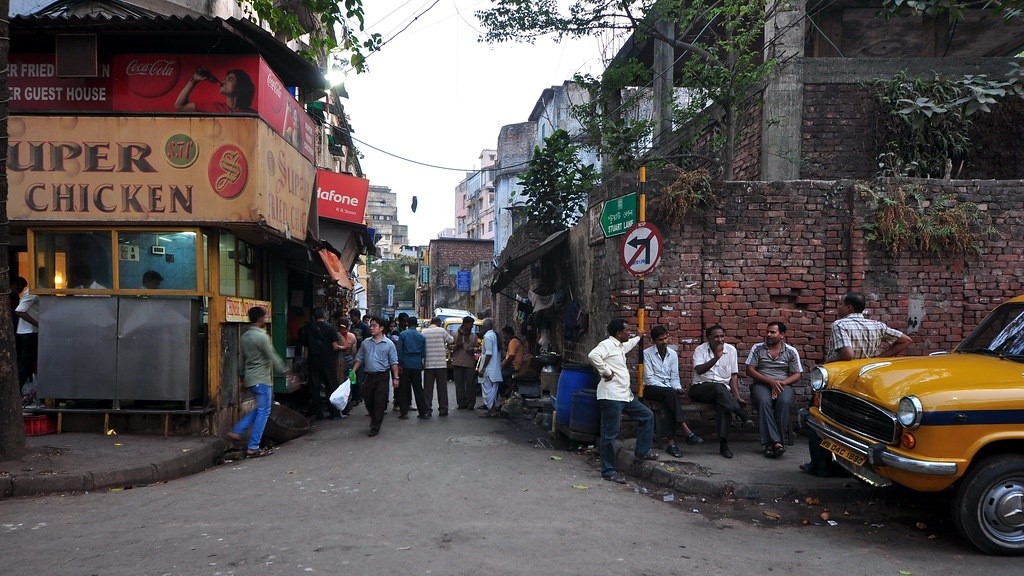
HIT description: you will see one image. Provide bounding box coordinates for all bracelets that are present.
[482,368,486,371]
[190,77,198,85]
[394,377,399,379]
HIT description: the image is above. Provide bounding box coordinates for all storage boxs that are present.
[23,414,54,435]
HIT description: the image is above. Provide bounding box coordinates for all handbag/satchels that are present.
[329,378,351,412]
[325,348,345,383]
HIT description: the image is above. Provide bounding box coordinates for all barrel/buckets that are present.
[557,361,599,434]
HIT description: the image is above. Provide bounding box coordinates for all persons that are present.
[643,326,704,458]
[141,271,164,289]
[688,325,754,458]
[303,306,454,437]
[745,321,804,458]
[283,101,302,151]
[588,319,659,485]
[10,277,27,330]
[174,68,256,113]
[475,318,503,418]
[225,307,291,458]
[15,267,48,403]
[477,326,523,410]
[798,293,913,477]
[72,269,107,289]
[447,316,482,410]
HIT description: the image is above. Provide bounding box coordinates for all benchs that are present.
[651,402,718,440]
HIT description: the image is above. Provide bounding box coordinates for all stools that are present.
[750,394,794,446]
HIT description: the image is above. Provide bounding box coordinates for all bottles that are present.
[349,368,357,385]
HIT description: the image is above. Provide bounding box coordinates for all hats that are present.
[502,326,515,335]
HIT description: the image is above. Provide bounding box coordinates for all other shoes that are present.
[764,450,775,458]
[775,444,788,458]
[419,407,499,418]
[720,446,733,458]
[367,428,379,436]
[364,405,419,419]
[685,434,704,445]
[744,420,755,432]
[799,463,810,472]
[641,451,659,460]
[667,444,682,457]
[602,469,626,483]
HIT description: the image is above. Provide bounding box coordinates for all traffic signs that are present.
[599,192,639,239]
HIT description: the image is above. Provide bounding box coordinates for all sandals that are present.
[224,435,249,445]
[246,448,274,458]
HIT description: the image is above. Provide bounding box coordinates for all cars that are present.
[414,305,485,380]
[793,294,1023,557]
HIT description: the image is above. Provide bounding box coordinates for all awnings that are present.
[484,231,568,302]
[293,247,352,291]
